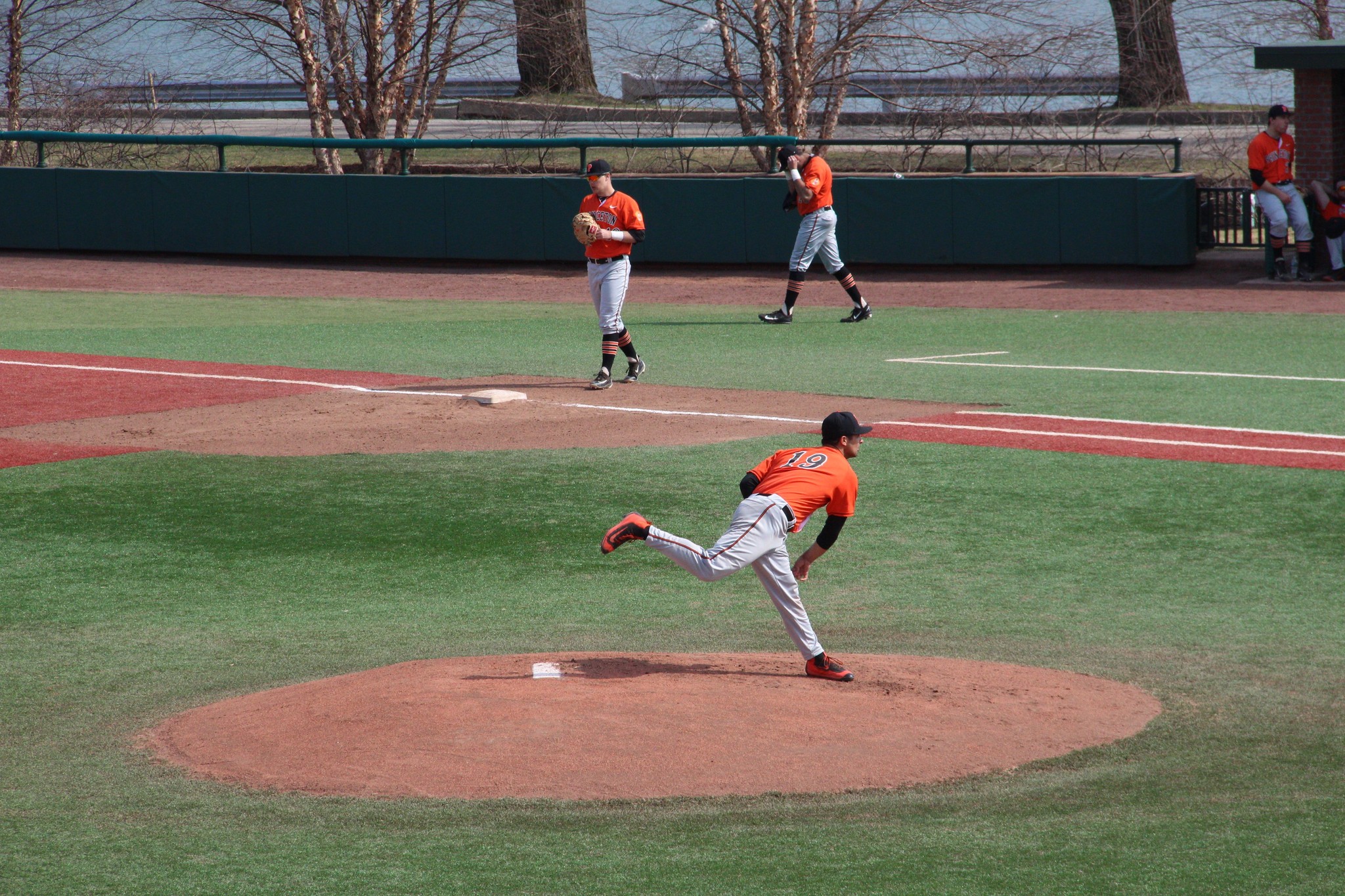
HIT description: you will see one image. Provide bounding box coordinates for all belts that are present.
[806,206,832,216]
[757,493,795,533]
[589,255,624,265]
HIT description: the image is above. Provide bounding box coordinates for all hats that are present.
[778,144,798,172]
[822,411,872,437]
[1269,105,1295,116]
[581,159,611,178]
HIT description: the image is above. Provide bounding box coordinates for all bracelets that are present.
[784,172,792,181]
[790,169,802,181]
[611,231,623,242]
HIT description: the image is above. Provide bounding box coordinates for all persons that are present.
[1247,105,1345,280]
[758,143,872,324]
[573,158,645,389]
[600,411,873,682]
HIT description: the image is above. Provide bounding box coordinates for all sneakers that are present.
[624,357,646,382]
[805,651,854,681]
[759,310,792,323]
[840,304,872,322]
[601,511,653,555]
[590,370,613,389]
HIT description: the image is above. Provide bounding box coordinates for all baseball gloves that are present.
[573,212,598,245]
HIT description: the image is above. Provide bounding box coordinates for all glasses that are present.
[587,173,607,182]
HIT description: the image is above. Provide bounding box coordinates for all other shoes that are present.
[1275,256,1286,278]
[1296,271,1313,282]
